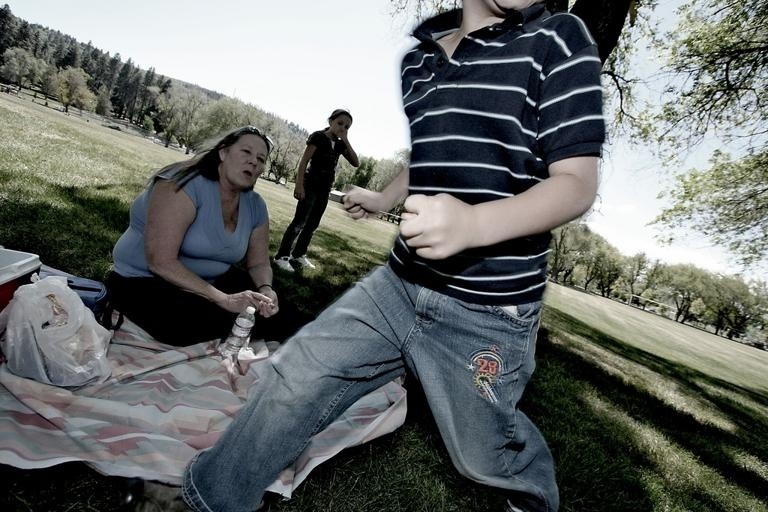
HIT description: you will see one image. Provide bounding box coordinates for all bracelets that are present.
[256,283,273,291]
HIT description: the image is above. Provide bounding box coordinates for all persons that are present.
[107,126,281,339]
[274,109,359,273]
[181,0,606,512]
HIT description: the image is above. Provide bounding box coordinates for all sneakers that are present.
[289,251,316,270]
[273,255,295,273]
[125,476,195,511]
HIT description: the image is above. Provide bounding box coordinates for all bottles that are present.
[221,306,256,357]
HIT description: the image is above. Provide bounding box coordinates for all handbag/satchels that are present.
[40,269,123,331]
